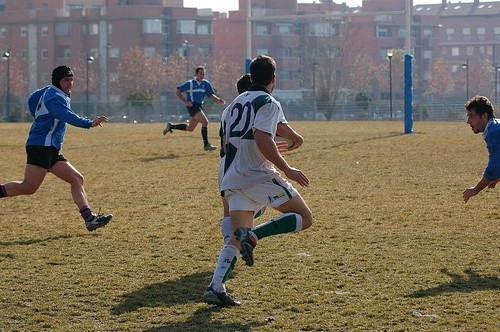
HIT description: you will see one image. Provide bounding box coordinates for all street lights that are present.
[387,48,393,121]
[462,59,468,102]
[86,52,95,118]
[2,49,10,122]
[312,63,320,121]
[183,39,189,80]
[494,64,500,105]
[106,43,113,107]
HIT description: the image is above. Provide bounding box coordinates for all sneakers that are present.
[163,123,174,136]
[202,287,242,307]
[86,208,113,232]
[204,143,217,150]
[233,226,257,267]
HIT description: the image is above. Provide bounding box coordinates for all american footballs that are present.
[274,134,294,148]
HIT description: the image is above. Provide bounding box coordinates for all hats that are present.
[52,65,74,93]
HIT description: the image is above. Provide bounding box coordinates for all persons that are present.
[219,75,303,283]
[204,55,311,306]
[0,65,114,233]
[462,96,500,202]
[163,66,225,150]
